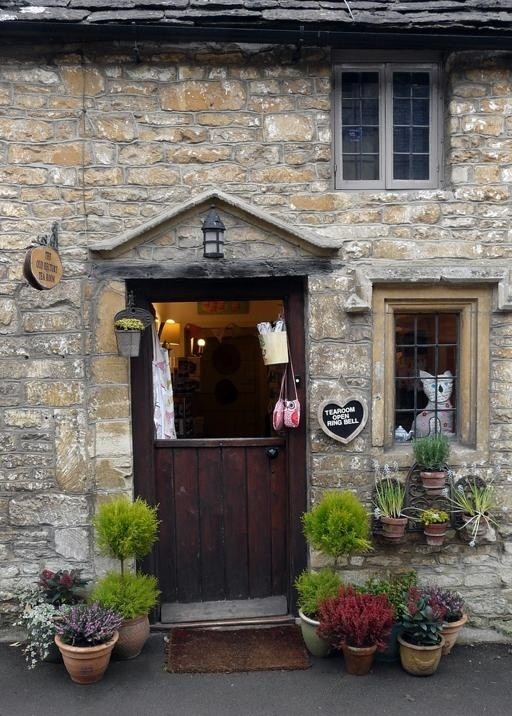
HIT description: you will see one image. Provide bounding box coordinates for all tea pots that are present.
[395,425,414,441]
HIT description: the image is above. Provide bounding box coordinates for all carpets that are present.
[165,628,312,673]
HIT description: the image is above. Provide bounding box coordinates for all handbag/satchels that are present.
[273,398,300,430]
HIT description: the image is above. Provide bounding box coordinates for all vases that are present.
[41,632,62,664]
[463,512,489,538]
[380,516,408,541]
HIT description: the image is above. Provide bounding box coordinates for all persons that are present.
[396,347,430,392]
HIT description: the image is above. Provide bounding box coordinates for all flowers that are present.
[34,569,92,609]
[441,464,508,547]
[370,458,439,521]
[399,586,448,646]
[43,600,124,648]
[424,579,466,624]
[9,588,71,670]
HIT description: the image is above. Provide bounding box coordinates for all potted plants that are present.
[420,509,449,546]
[88,495,162,659]
[364,567,417,663]
[42,601,124,684]
[315,585,394,675]
[112,318,145,357]
[410,432,451,496]
[293,489,374,659]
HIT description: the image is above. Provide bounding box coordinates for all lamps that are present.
[157,319,180,349]
[201,204,225,258]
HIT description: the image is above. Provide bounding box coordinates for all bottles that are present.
[178,360,189,370]
